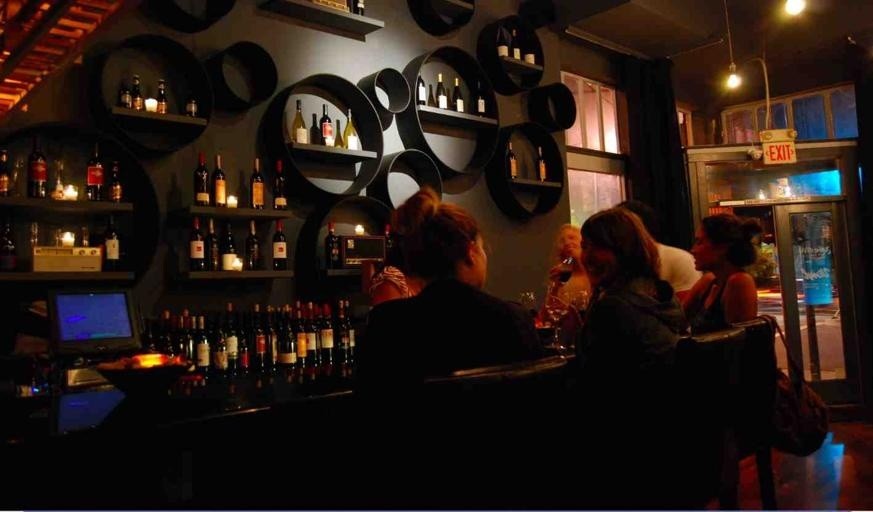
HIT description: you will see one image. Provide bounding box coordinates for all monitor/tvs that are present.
[47,287,143,356]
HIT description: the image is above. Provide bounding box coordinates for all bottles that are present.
[436,74,446,110]
[319,103,333,147]
[452,78,464,112]
[120,75,199,119]
[323,221,340,269]
[25,214,120,272]
[427,84,435,107]
[446,89,452,110]
[497,26,535,65]
[334,119,343,149]
[292,99,307,144]
[473,79,485,117]
[416,70,425,105]
[507,142,516,179]
[0,223,16,272]
[157,299,357,384]
[343,110,358,151]
[309,113,319,144]
[0,138,122,203]
[271,221,286,272]
[194,152,287,209]
[383,225,392,259]
[190,217,258,271]
[536,147,546,182]
[352,1,363,15]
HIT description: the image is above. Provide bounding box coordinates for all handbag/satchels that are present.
[758,314,828,456]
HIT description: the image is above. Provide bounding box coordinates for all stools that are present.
[154,314,784,509]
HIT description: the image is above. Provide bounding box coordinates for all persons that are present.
[544,198,760,377]
[367,257,424,308]
[346,187,543,397]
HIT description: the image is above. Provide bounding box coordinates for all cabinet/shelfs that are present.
[1,0,580,287]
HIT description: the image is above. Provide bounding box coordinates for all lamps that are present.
[723,0,742,89]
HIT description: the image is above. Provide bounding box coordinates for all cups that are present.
[557,329,575,359]
[535,327,555,345]
[519,288,536,310]
[559,255,574,282]
